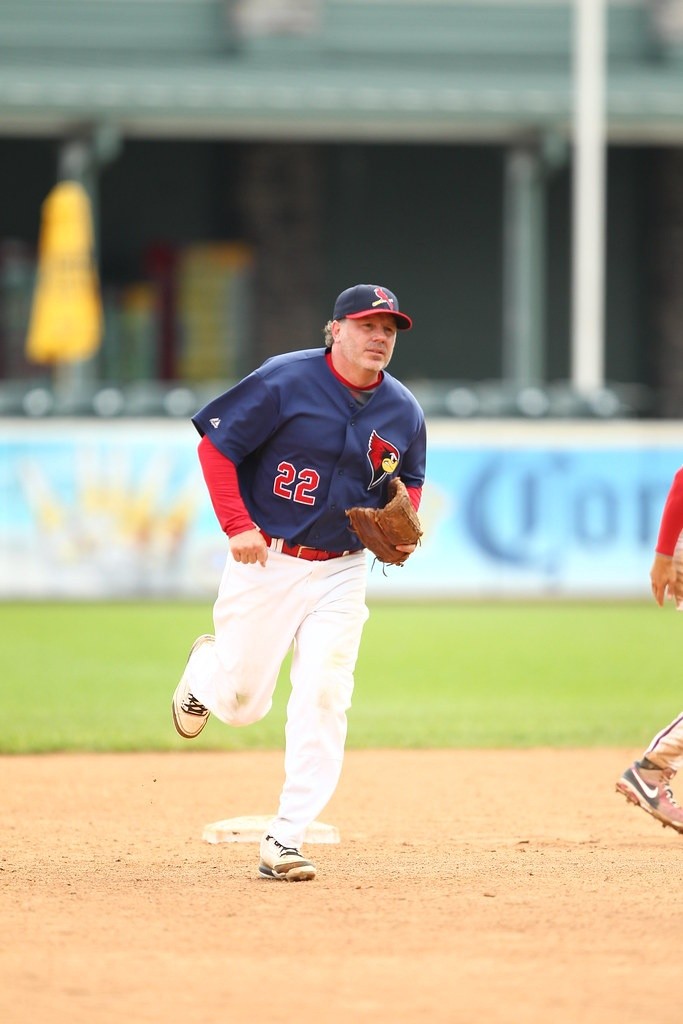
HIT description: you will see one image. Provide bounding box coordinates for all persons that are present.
[614,465,683,835]
[172,283,427,881]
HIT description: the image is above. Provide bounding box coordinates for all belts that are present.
[257,529,363,561]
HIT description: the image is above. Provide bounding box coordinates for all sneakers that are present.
[614,762,682,834]
[258,832,316,881]
[172,634,211,738]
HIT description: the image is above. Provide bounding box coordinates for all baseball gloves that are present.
[343,475,424,577]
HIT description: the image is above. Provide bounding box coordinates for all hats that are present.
[332,284,412,331]
